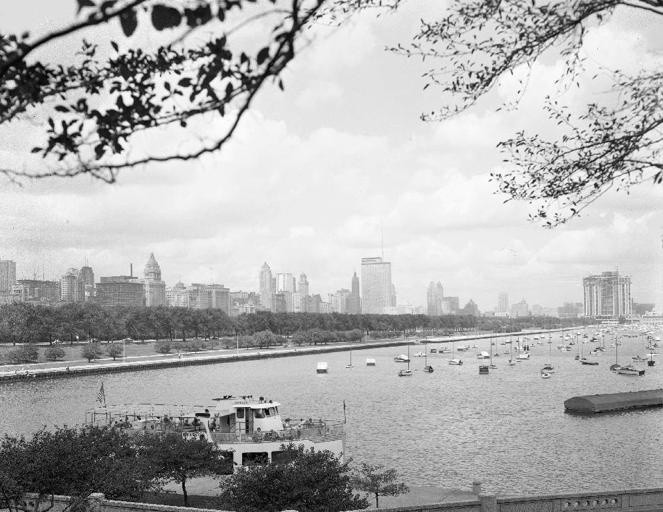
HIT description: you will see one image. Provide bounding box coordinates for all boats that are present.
[479,364,489,374]
[476,350,490,359]
[394,354,411,362]
[457,343,479,352]
[366,357,376,366]
[559,319,662,376]
[414,347,444,358]
[82,380,347,477]
[541,363,556,378]
[316,361,329,374]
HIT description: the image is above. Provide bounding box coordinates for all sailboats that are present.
[422,334,434,373]
[399,336,413,377]
[488,331,498,369]
[345,351,355,368]
[500,334,530,368]
[530,328,555,348]
[448,337,463,366]
